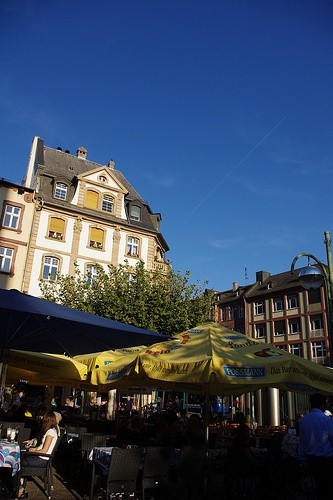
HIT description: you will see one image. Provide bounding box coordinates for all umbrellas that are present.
[0,349,89,402]
[91,320,333,500]
[0,288,179,358]
[31,345,157,396]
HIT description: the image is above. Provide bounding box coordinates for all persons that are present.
[299,393,333,500]
[0,389,333,466]
[12,410,60,498]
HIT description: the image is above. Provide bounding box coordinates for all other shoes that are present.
[19,491,28,499]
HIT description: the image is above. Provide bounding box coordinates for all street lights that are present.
[290,232,333,367]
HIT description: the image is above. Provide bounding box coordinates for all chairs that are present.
[0,421,208,500]
[224,438,277,500]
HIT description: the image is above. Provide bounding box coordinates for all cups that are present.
[6,427,16,441]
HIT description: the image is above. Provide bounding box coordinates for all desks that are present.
[0,438,21,477]
